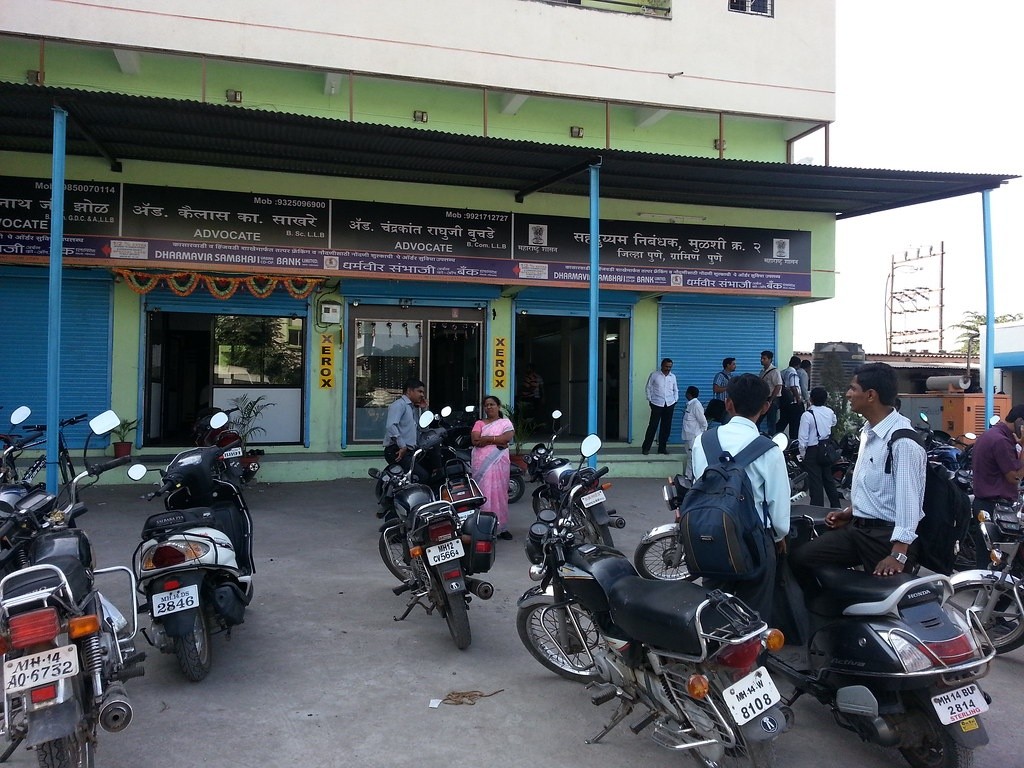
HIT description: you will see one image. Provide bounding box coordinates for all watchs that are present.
[890,551,907,565]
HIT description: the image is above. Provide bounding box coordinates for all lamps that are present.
[571,127,583,137]
[413,110,428,122]
[714,139,725,149]
[26,70,46,84]
[227,89,242,102]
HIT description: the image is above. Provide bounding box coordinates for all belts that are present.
[851,516,894,529]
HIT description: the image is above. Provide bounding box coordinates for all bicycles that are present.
[0,413,89,504]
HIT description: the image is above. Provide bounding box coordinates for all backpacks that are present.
[885,428,972,575]
[680,424,779,580]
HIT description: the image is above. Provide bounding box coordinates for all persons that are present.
[692,373,790,672]
[382,378,429,484]
[520,363,543,418]
[969,404,1024,629]
[776,356,811,438]
[702,358,736,429]
[642,358,679,454]
[471,396,515,540]
[682,386,708,479]
[797,387,841,509]
[758,351,783,435]
[793,364,928,585]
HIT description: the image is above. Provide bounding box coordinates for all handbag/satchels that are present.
[704,398,727,419]
[817,438,837,466]
[777,387,794,409]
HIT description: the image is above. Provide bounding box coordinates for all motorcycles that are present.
[368,405,535,649]
[634,413,1024,768]
[1,405,148,768]
[516,433,788,768]
[127,406,257,682]
[521,409,626,550]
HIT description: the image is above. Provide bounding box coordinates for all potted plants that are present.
[106,418,148,456]
[226,394,279,463]
[497,401,544,475]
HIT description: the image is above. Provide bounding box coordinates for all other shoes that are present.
[498,531,512,540]
[642,450,649,455]
[997,617,1018,631]
[658,449,668,454]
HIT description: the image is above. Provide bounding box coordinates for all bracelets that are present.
[493,436,496,442]
[490,442,493,445]
